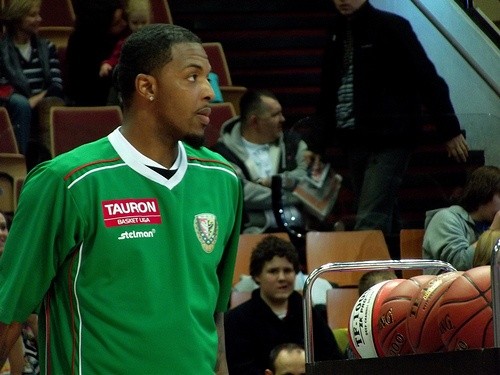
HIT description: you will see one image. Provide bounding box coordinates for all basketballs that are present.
[348,278,407,358]
[377,275,437,357]
[405,271,466,354]
[438,265,495,351]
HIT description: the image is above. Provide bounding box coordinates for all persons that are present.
[209,89,316,233]
[0,24,244,375]
[224,234,341,375]
[309,0,471,240]
[265,343,305,375]
[0,0,154,375]
[344,166,500,361]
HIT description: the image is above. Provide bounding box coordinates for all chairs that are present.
[0,0,425,330]
